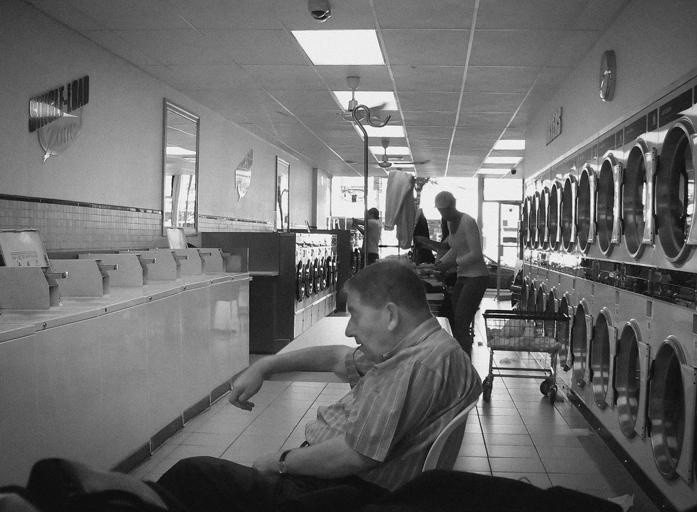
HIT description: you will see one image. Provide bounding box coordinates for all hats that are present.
[435,191,454,209]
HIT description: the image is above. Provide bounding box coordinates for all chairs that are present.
[415,394,480,477]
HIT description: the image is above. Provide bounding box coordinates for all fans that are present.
[304,75,431,169]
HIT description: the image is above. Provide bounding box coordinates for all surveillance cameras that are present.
[511,169,517,174]
[310,7,329,19]
[314,13,331,22]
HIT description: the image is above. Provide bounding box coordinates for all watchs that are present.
[276,449,293,480]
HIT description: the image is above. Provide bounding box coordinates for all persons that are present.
[431,191,490,362]
[414,215,459,337]
[351,208,383,269]
[155,258,482,510]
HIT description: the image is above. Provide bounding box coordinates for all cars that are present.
[385,253,517,291]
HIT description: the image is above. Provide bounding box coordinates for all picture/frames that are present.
[271,152,292,233]
[160,97,199,237]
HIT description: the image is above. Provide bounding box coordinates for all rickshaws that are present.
[481,309,571,401]
